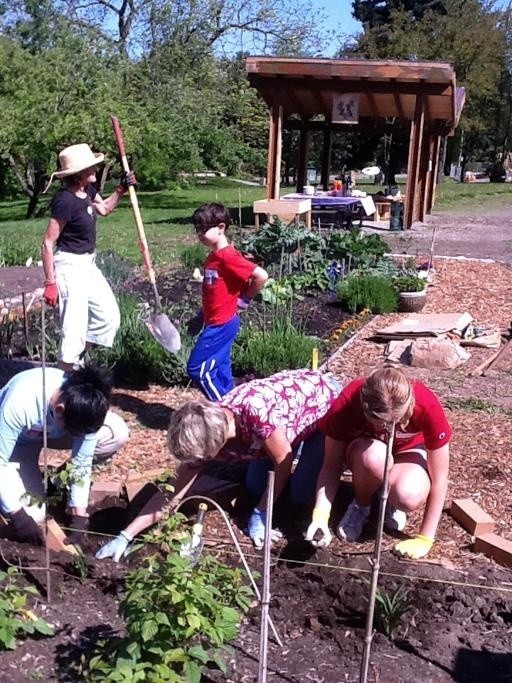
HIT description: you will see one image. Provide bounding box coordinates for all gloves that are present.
[116,171,137,195]
[62,514,89,546]
[9,508,44,548]
[237,294,251,310]
[43,280,60,308]
[248,508,283,550]
[305,508,331,548]
[392,533,435,560]
[185,311,204,337]
[95,531,132,562]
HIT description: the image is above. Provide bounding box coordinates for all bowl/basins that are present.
[302,186,315,195]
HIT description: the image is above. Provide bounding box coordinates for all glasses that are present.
[195,224,215,235]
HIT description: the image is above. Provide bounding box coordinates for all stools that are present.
[374,202,391,221]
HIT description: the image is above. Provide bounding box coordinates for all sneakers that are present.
[385,507,407,531]
[337,500,371,542]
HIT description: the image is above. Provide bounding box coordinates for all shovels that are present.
[180,503,208,570]
[111,117,182,354]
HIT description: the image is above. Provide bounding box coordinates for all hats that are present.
[53,143,105,178]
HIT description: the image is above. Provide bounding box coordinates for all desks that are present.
[253,198,311,231]
[280,193,376,230]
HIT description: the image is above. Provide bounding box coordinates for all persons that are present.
[42,143,137,368]
[184,202,268,403]
[1,367,129,545]
[95,368,343,562]
[305,365,452,560]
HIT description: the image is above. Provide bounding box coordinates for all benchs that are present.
[311,209,338,229]
